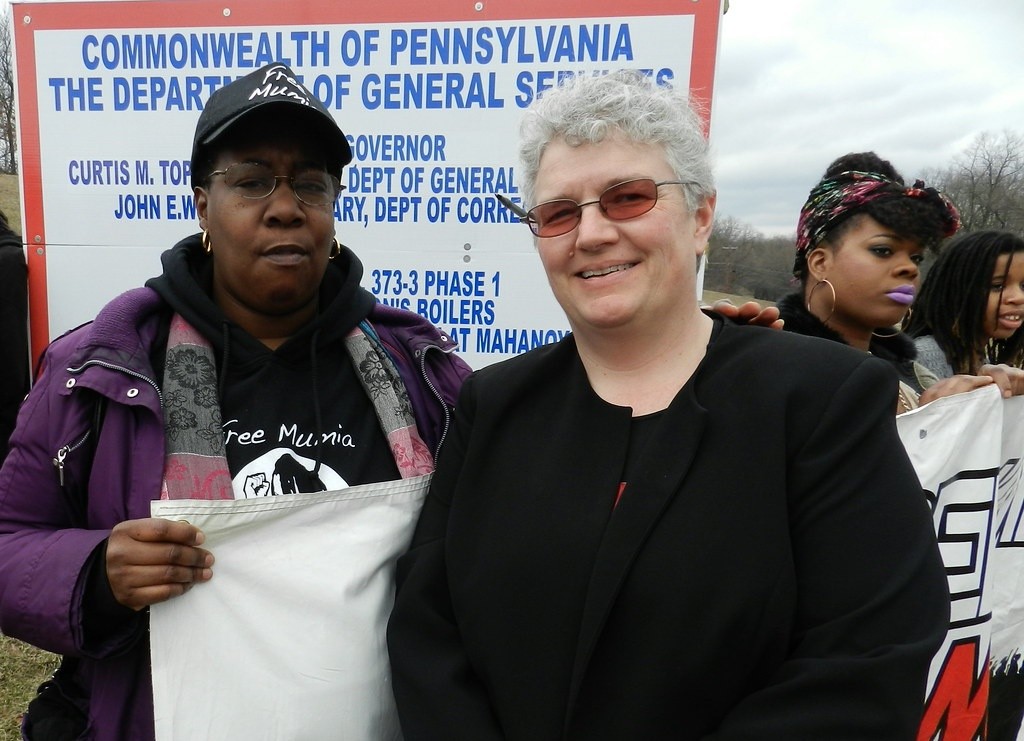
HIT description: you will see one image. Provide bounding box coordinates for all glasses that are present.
[201,161,346,206]
[520,179,699,238]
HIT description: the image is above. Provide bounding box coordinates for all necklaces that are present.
[897,391,912,414]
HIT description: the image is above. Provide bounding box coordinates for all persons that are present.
[0,58,787,739]
[772,152,1023,421]
[0,207,32,469]
[386,69,947,739]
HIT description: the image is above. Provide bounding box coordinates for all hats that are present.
[190,62,352,178]
